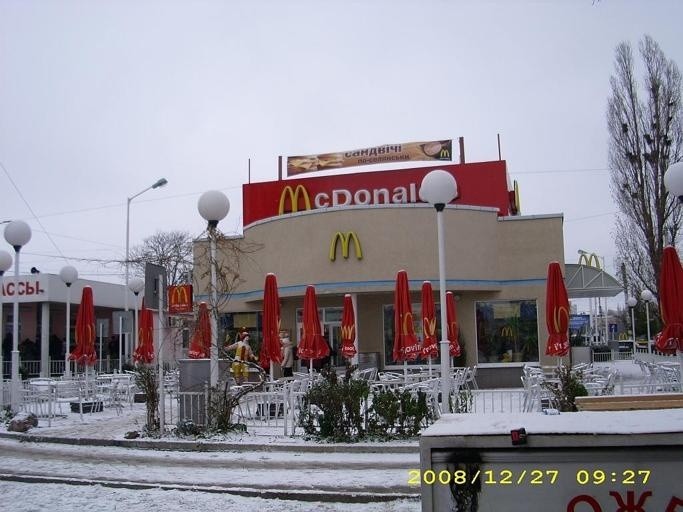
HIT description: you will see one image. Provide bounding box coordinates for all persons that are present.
[126,353,133,366]
[279,331,295,382]
[223,331,258,385]
[301,336,334,372]
[104,336,119,370]
[1,332,69,383]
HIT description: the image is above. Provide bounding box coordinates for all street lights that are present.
[61,264,78,382]
[124,177,168,371]
[0,249,13,412]
[627,296,638,364]
[641,290,652,355]
[198,189,229,427]
[421,170,458,414]
[4,220,31,417]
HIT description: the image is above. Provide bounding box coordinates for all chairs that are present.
[518,357,678,420]
[224,358,481,433]
[3,358,177,431]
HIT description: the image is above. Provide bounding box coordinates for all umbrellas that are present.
[655,246,683,355]
[544,258,572,379]
